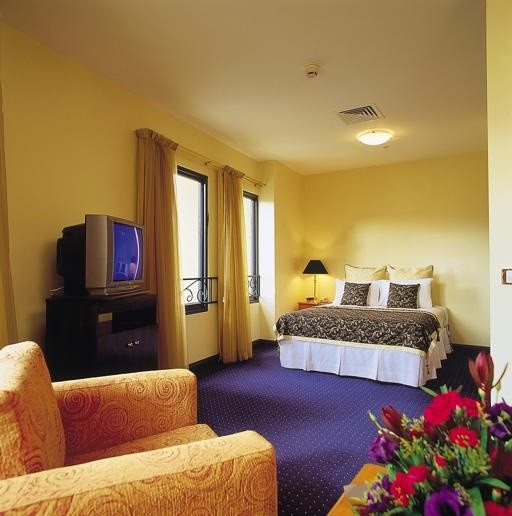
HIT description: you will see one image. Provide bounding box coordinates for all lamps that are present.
[303,260,328,300]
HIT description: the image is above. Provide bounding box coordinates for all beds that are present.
[277,305,448,388]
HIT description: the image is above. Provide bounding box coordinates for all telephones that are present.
[320,297,329,304]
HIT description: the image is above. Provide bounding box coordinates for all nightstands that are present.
[298,302,329,310]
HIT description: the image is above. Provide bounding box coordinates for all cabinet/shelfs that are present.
[44,294,157,382]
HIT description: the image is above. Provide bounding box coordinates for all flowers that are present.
[344,351,511,516]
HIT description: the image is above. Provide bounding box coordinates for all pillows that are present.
[331,264,433,309]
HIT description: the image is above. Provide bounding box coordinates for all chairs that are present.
[1,341,277,516]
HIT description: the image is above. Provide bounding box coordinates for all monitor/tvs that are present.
[55,213,147,298]
